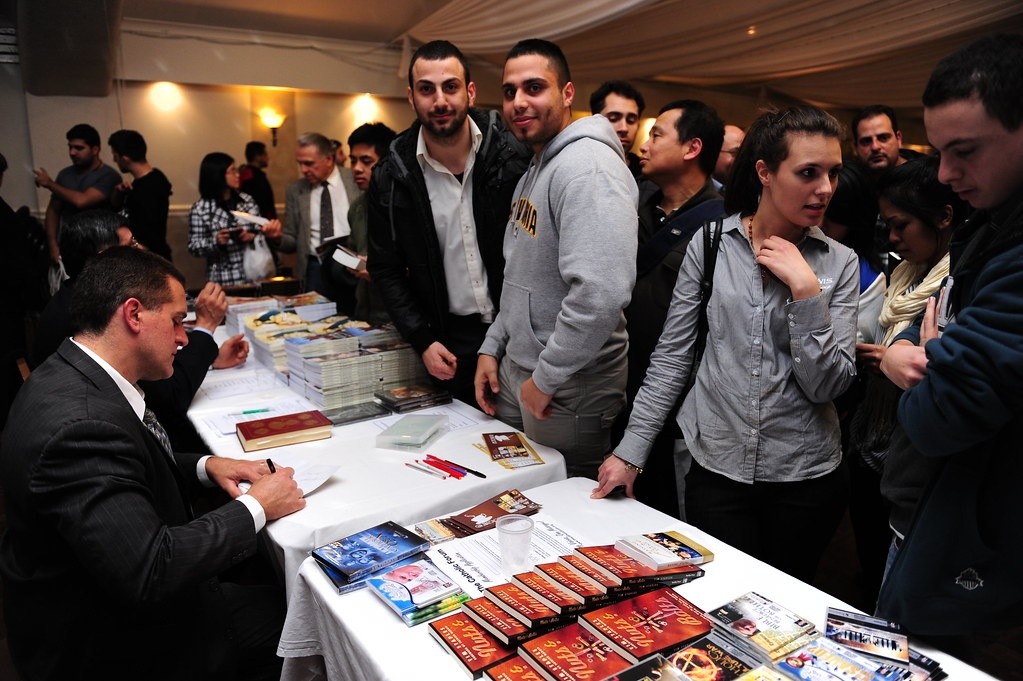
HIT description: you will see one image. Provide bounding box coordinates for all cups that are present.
[495,514,534,580]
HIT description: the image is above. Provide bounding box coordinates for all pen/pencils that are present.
[210,263,216,280]
[415,454,467,480]
[266,458,276,473]
[405,463,446,480]
[229,409,274,415]
[444,460,486,478]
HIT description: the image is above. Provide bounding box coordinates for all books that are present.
[310,522,949,680]
[332,244,366,271]
[236,410,334,453]
[226,292,452,426]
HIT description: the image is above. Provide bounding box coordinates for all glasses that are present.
[128,236,138,249]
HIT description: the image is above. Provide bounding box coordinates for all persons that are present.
[237,141,279,262]
[187,156,283,287]
[473,36,640,478]
[367,39,533,410]
[827,108,955,612]
[0,248,306,681]
[24,215,249,433]
[729,598,790,635]
[877,39,1023,681]
[261,121,399,328]
[588,80,745,524]
[383,561,445,595]
[591,100,860,589]
[0,123,173,411]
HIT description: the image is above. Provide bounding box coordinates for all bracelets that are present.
[625,464,643,473]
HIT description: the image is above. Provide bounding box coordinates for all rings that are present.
[260,462,265,465]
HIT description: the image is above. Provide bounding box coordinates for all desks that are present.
[168,293,1005,681]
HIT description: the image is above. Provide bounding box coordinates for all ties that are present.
[320,181,334,239]
[143,408,176,465]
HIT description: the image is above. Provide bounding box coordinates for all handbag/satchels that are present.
[243,234,276,283]
[686,217,723,386]
